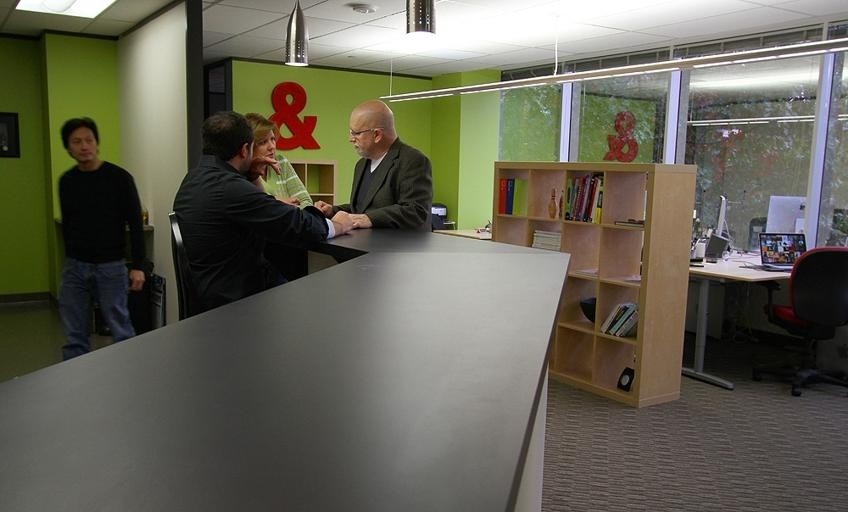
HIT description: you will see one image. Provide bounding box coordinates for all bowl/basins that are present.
[580,298,596,324]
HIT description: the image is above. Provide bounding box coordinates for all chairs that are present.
[745,244,847,402]
[167,211,194,321]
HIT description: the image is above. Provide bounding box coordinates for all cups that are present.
[721,251,729,262]
[696,243,705,258]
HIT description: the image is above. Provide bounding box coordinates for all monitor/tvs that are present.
[766,195,834,242]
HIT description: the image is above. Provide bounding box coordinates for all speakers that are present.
[705,233,730,258]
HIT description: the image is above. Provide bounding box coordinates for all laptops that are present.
[758,232,807,270]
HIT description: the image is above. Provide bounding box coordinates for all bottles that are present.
[141,207,148,226]
[548,186,558,218]
[694,218,702,237]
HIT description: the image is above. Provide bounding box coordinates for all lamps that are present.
[282,0,309,69]
[403,0,438,39]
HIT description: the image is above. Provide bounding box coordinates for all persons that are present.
[314,100,433,232]
[173,111,354,316]
[58,119,145,361]
[245,113,314,211]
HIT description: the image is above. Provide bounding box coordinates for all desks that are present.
[431,227,492,241]
[682,244,794,399]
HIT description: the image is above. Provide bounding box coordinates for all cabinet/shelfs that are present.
[290,156,340,214]
[489,157,702,411]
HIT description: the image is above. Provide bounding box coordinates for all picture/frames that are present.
[0,110,22,159]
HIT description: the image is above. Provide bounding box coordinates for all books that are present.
[600,302,640,337]
[499,173,604,252]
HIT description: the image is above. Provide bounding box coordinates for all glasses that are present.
[350,128,385,137]
